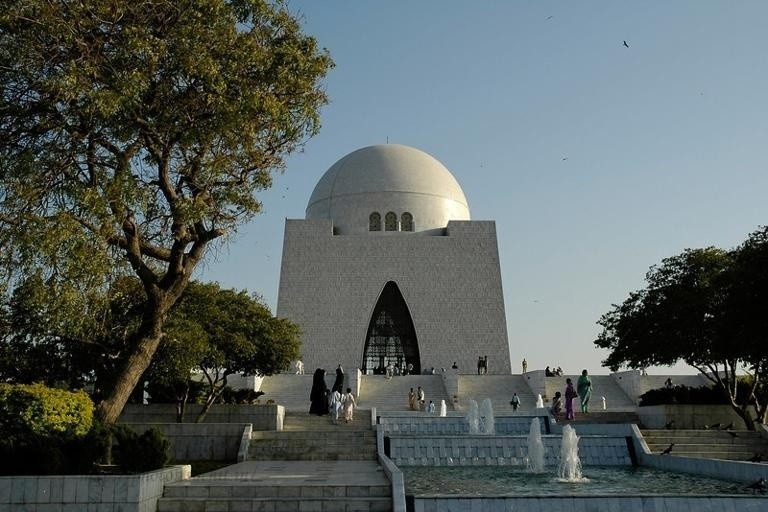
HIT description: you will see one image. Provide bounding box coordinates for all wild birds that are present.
[622,41,629,48]
[660,443,674,455]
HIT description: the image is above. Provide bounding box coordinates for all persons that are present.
[545,366,564,377]
[423,362,458,375]
[296,359,356,424]
[386,362,394,379]
[536,394,544,408]
[408,360,414,374]
[565,378,575,421]
[511,393,520,412]
[552,392,561,422]
[478,356,488,375]
[522,358,528,373]
[408,387,447,417]
[577,369,593,414]
[665,376,672,388]
